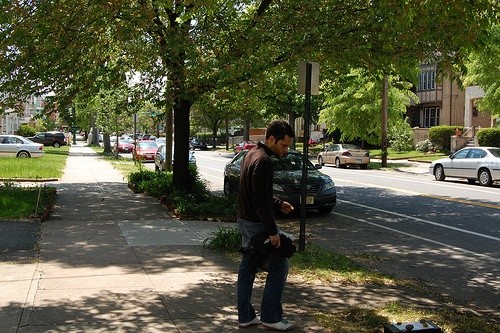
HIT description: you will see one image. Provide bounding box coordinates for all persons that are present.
[236,120,297,330]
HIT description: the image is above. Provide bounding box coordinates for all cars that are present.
[429,146,500,188]
[98,131,174,154]
[232,140,258,155]
[154,143,196,172]
[308,132,324,145]
[0,135,44,159]
[316,144,370,169]
[223,147,337,215]
[189,136,208,151]
[132,140,159,163]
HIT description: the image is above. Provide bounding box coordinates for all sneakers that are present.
[262,319,293,331]
[238,316,261,328]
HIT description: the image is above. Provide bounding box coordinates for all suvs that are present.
[25,131,68,148]
